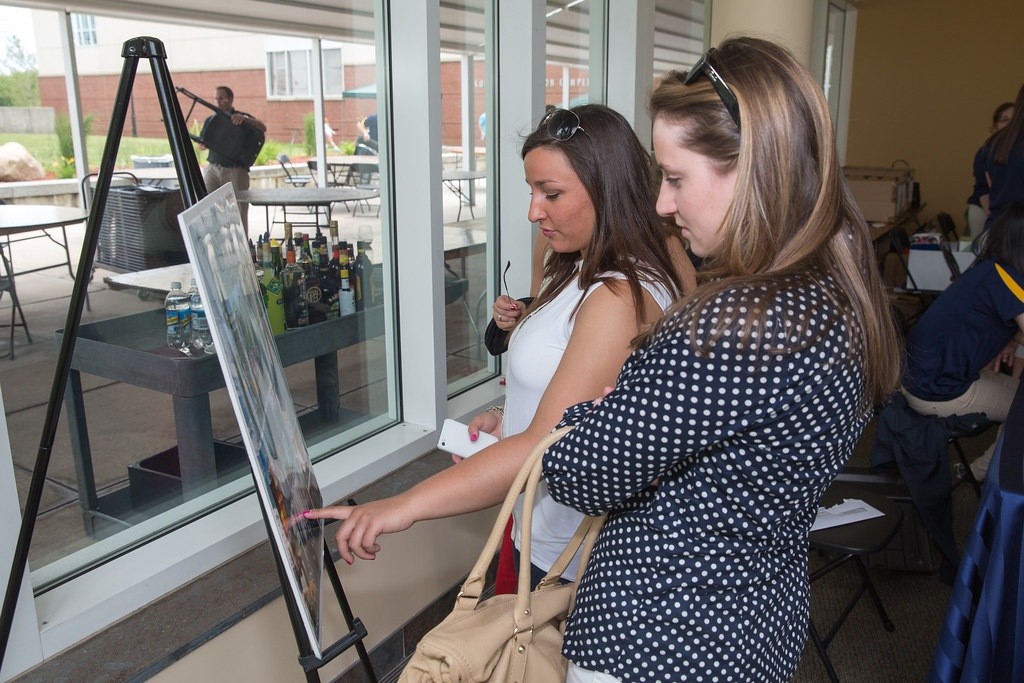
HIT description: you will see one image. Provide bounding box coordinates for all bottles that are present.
[165,281,191,349]
[247,220,376,337]
[189,279,215,354]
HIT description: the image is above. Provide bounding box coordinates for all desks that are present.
[248,183,379,240]
[54,268,470,535]
[0,205,90,360]
[309,155,380,212]
[442,169,485,221]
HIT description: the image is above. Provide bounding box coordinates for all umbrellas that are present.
[341,83,376,98]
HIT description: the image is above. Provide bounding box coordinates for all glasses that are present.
[538,107,592,142]
[997,115,1013,125]
[215,96,230,102]
[682,47,741,131]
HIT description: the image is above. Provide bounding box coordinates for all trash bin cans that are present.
[133,156,171,169]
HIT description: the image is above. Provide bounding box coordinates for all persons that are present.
[542,35,902,683]
[900,85,1024,683]
[199,86,266,242]
[303,102,684,633]
[479,113,486,140]
[324,118,340,150]
[352,112,378,155]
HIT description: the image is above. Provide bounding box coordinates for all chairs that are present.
[943,247,965,280]
[871,308,991,580]
[937,211,959,243]
[875,241,938,331]
[159,84,265,175]
[277,153,380,218]
[803,487,900,683]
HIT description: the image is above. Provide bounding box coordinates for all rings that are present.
[498,315,502,321]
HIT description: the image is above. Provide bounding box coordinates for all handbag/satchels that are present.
[398,426,607,683]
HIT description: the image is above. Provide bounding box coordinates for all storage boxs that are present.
[904,243,974,291]
[128,436,246,502]
[843,167,914,225]
